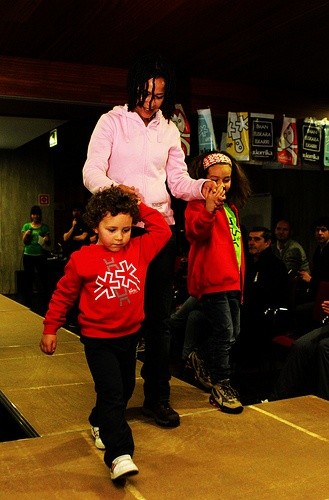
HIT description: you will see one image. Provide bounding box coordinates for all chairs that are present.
[271,279,329,361]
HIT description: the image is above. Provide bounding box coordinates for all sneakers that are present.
[187,352,213,389]
[141,398,179,428]
[90,426,105,449]
[209,379,243,414]
[110,454,139,479]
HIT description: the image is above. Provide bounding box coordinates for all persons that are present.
[40,183,173,482]
[21,205,51,303]
[61,204,329,402]
[187,149,245,414]
[82,67,226,429]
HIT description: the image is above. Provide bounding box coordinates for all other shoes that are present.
[137,343,145,353]
